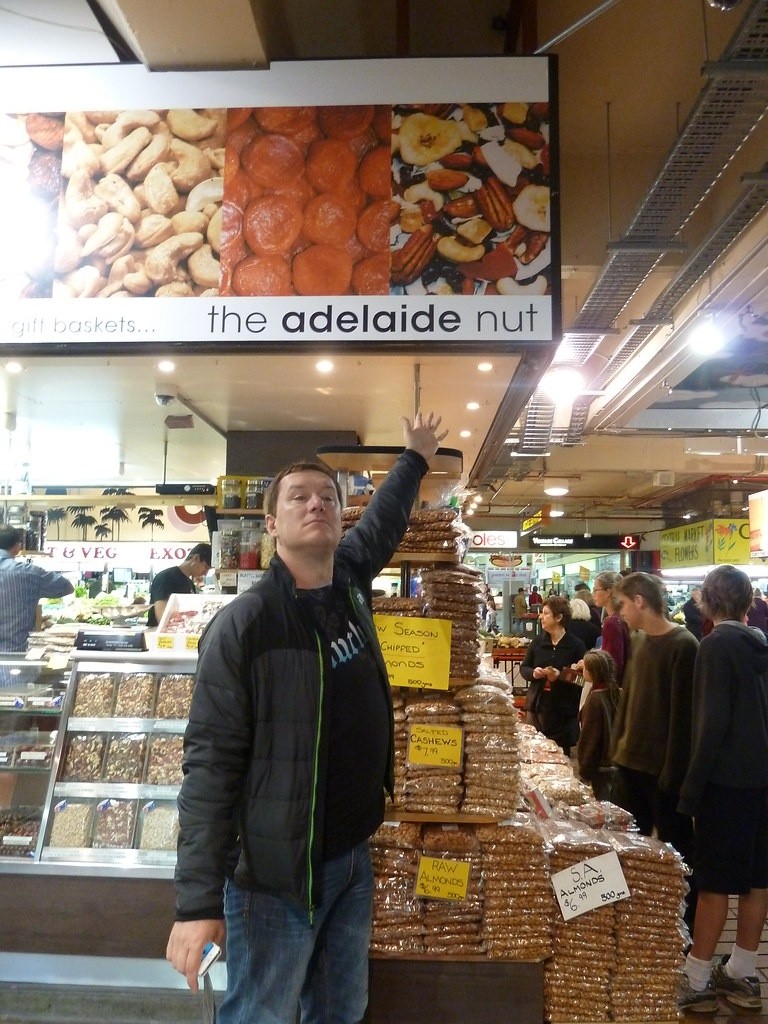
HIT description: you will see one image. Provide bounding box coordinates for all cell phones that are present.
[199,938,222,979]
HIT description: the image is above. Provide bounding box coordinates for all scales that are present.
[74,603,154,651]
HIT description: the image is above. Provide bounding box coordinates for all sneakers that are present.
[709,953,762,1009]
[675,972,719,1012]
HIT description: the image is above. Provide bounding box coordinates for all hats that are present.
[518,588,525,592]
[752,588,761,596]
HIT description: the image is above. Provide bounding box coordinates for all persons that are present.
[481,564,768,1013]
[165,411,450,1024]
[146,543,211,627]
[0,524,74,652]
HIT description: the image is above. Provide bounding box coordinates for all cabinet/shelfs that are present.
[213,509,273,574]
[660,520,749,567]
[313,446,548,1024]
[0,651,206,864]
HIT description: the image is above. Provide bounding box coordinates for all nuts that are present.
[391,100,553,300]
[1,107,226,302]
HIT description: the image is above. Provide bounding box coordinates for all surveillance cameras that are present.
[153,384,179,408]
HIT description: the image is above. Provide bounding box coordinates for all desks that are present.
[492,656,529,688]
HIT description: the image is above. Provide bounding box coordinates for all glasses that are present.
[593,587,607,594]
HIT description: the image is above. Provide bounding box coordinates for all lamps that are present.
[550,504,564,517]
[653,472,675,486]
[466,492,482,515]
[543,479,570,496]
